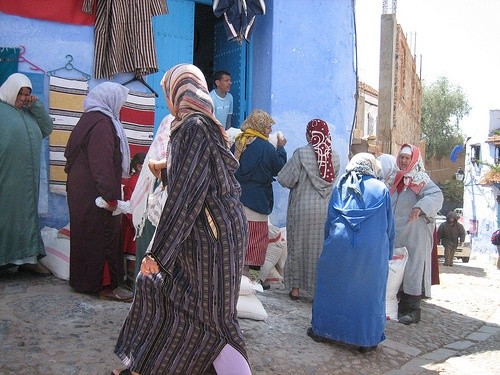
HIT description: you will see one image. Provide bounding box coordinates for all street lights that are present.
[455,167,465,207]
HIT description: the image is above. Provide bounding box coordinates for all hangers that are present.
[120,72,159,98]
[46,54,92,81]
[17,45,46,75]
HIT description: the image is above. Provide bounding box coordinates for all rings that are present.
[145,269,150,274]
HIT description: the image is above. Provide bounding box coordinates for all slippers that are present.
[289,290,298,300]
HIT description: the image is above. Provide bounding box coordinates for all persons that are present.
[228,109,287,290]
[276,118,341,303]
[307,152,396,354]
[376,143,500,324]
[111,62,253,375]
[63,81,176,302]
[209,70,234,131]
[0,73,53,281]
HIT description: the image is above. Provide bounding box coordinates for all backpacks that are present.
[491,230,500,244]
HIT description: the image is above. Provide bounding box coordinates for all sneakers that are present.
[99,287,134,301]
[17,263,51,277]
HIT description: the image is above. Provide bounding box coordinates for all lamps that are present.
[454,167,470,186]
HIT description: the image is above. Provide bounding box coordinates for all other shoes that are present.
[110,367,139,375]
[360,344,377,354]
[257,280,270,290]
[449,262,453,266]
[443,262,448,266]
[307,327,320,341]
[398,311,419,325]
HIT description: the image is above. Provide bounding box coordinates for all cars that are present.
[454,207,464,216]
[435,216,473,262]
